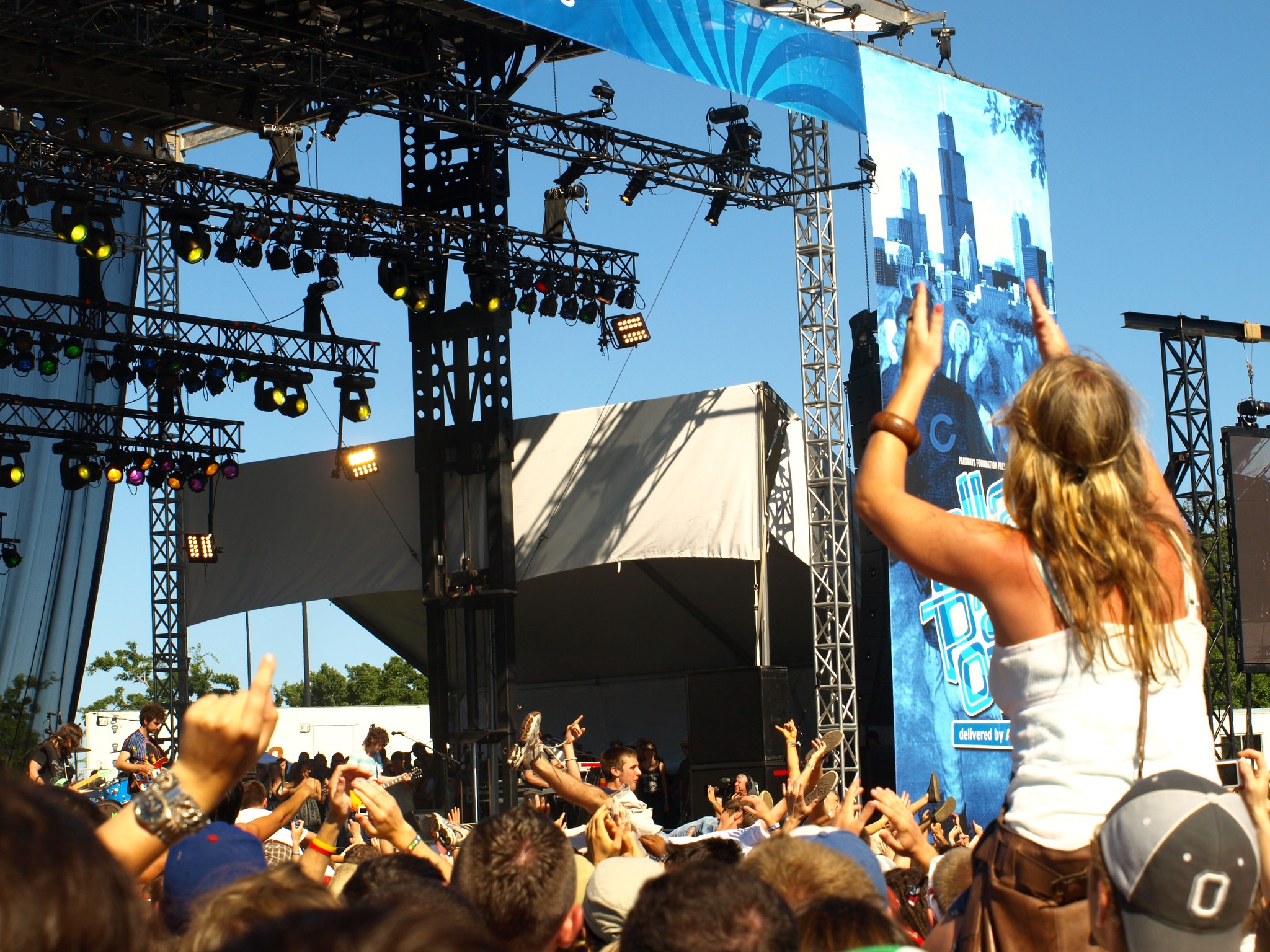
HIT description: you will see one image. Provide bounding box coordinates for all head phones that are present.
[742,773,752,790]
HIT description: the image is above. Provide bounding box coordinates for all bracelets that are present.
[712,801,717,806]
[804,764,815,771]
[291,852,299,855]
[660,852,669,862]
[664,796,668,798]
[152,771,209,834]
[786,739,801,747]
[665,802,668,803]
[405,834,422,854]
[288,788,291,793]
[449,846,460,852]
[828,810,833,819]
[307,838,337,857]
[868,413,923,457]
[564,757,578,760]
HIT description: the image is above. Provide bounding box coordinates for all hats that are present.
[164,820,269,934]
[1101,766,1259,952]
[786,825,888,914]
[582,856,667,944]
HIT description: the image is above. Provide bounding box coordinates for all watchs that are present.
[767,822,781,831]
[133,785,182,847]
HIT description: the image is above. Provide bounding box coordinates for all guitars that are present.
[131,756,170,794]
[348,767,422,813]
[53,769,111,793]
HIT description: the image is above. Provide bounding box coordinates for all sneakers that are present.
[931,797,956,823]
[429,812,473,850]
[506,710,569,772]
[927,772,941,804]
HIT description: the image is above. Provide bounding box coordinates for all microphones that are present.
[58,708,63,725]
[44,729,54,735]
[391,732,405,735]
[96,716,100,726]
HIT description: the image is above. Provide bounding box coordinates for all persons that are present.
[0,653,1270,952]
[855,279,1225,952]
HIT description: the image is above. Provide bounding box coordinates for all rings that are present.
[906,316,912,323]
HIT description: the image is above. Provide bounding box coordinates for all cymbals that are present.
[67,746,92,753]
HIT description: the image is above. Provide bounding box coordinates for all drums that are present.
[99,776,133,807]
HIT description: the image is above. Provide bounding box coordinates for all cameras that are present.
[359,806,368,815]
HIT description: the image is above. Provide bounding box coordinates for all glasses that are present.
[378,743,387,748]
[681,745,689,749]
[643,747,654,752]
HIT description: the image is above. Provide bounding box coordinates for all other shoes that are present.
[805,771,839,807]
[805,730,846,765]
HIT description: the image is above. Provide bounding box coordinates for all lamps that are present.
[589,78,615,107]
[748,120,762,145]
[709,105,749,124]
[854,153,877,180]
[96,716,108,726]
[1237,397,1270,428]
[0,432,379,492]
[705,192,729,226]
[0,543,23,569]
[0,161,651,422]
[186,533,218,564]
